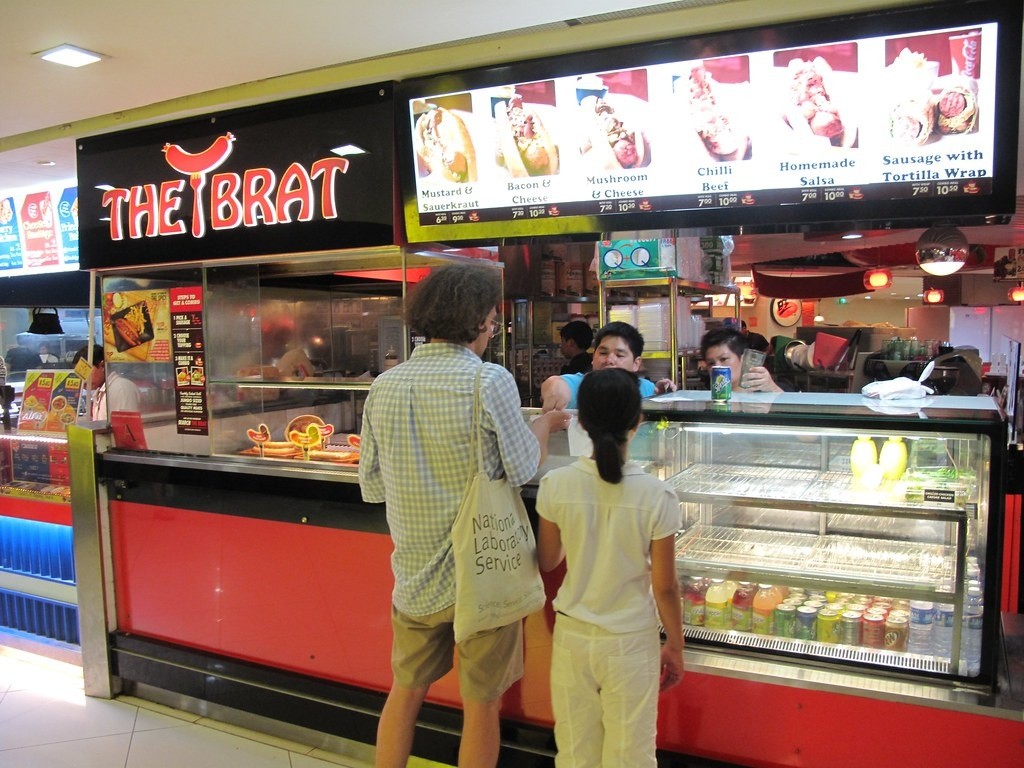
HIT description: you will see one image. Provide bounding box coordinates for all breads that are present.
[253,441,351,459]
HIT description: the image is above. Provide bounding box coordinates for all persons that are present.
[69,342,142,422]
[37,341,60,364]
[762,334,777,374]
[5,334,42,383]
[741,320,769,350]
[356,259,573,768]
[995,249,1016,277]
[534,367,686,768]
[703,400,773,416]
[698,327,795,394]
[540,322,677,414]
[556,318,597,377]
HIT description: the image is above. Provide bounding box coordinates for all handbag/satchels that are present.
[450,363,546,645]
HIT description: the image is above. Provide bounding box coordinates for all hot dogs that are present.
[581,91,644,170]
[673,68,749,161]
[114,318,140,346]
[493,95,557,179]
[780,57,857,148]
[414,107,476,183]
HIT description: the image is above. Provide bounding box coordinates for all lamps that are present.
[916,224,971,277]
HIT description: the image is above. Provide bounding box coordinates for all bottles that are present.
[989,352,1006,374]
[677,572,785,638]
[384,346,397,368]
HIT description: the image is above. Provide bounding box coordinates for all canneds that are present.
[775,586,911,652]
[712,365,731,404]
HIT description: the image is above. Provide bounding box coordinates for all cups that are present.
[880,339,953,360]
[32,415,46,428]
[60,414,73,430]
[51,396,66,416]
[739,347,764,388]
[24,401,37,415]
[946,32,981,80]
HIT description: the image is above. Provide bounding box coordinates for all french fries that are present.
[123,305,147,335]
